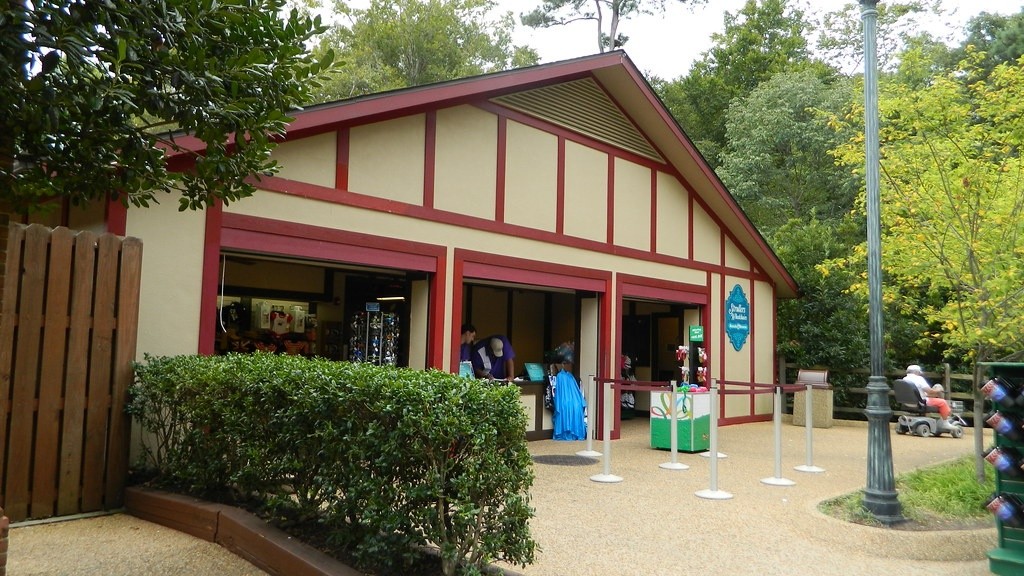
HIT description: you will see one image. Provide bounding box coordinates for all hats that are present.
[491,338,503,357]
[908,364,923,374]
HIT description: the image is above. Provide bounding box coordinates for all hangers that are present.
[271,305,284,313]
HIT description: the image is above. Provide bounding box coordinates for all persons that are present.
[473,335,515,381]
[461,323,476,362]
[903,365,956,429]
[621,352,636,409]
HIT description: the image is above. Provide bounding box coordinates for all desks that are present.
[650,392,710,451]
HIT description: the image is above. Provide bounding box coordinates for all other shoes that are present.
[942,424,959,430]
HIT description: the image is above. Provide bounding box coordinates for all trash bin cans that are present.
[793,368,834,429]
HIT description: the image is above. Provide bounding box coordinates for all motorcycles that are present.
[894,379,967,438]
